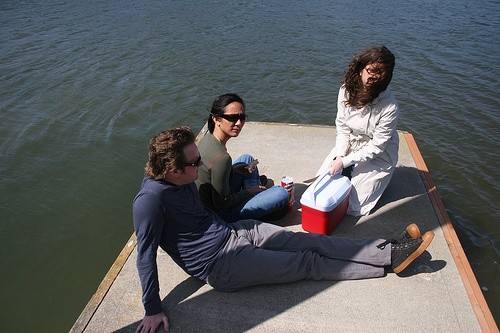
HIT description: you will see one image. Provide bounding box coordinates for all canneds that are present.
[281,176,295,206]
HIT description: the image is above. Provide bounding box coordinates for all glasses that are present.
[175,156,201,167]
[214,113,246,122]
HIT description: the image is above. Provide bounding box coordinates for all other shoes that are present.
[260,175,267,185]
[391,223,435,273]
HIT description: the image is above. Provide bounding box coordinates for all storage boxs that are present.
[300,171,353,234]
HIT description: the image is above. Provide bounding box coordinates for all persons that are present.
[302,45,399,217]
[132,126,435,333]
[193,92,294,221]
